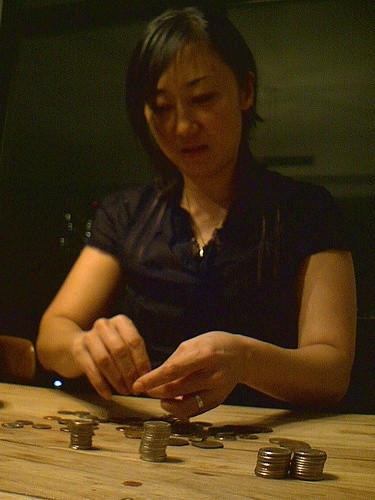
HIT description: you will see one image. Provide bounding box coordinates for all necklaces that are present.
[185,189,210,257]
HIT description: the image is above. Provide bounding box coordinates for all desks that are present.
[0,383,375,500]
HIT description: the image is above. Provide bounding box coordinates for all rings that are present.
[193,393,204,411]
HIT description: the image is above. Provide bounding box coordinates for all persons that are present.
[34,8,357,419]
